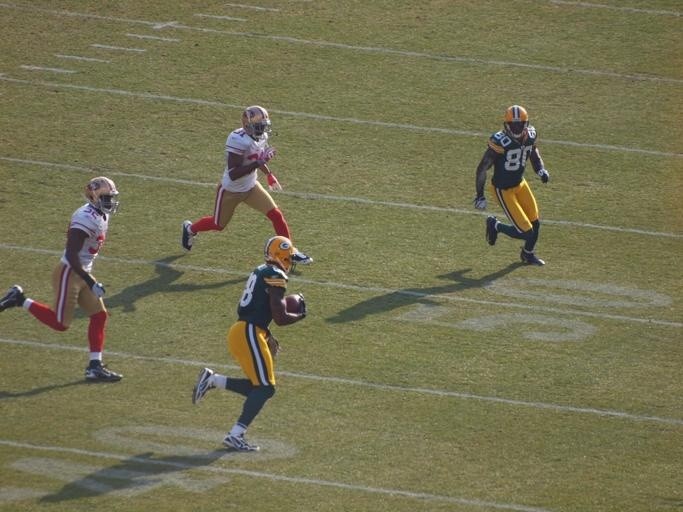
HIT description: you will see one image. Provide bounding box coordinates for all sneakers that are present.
[86,363,124,381]
[519,248,545,265]
[191,367,216,405]
[0,285,23,311]
[486,216,498,245]
[222,432,260,451]
[291,250,312,264]
[182,219,196,249]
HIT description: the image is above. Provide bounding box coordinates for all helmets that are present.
[264,236,294,273]
[84,176,119,212]
[242,105,272,138]
[504,105,529,137]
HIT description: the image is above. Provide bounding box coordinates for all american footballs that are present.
[285,292,303,315]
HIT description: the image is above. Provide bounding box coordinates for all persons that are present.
[180,104,315,267]
[0,175,124,382]
[190,234,310,453]
[473,104,550,267]
